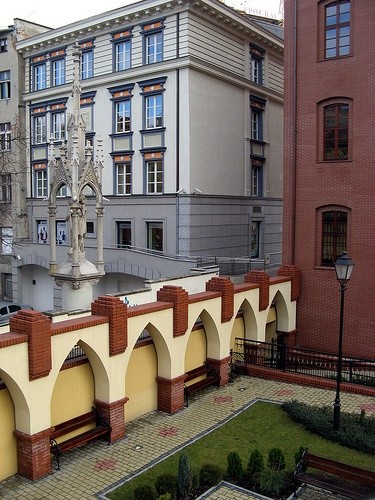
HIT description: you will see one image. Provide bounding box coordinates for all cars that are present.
[0,301,33,326]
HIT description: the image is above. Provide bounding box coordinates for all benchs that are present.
[183,360,222,407]
[293,447,375,500]
[49,406,112,471]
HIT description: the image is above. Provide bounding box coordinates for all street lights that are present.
[333,251,354,431]
